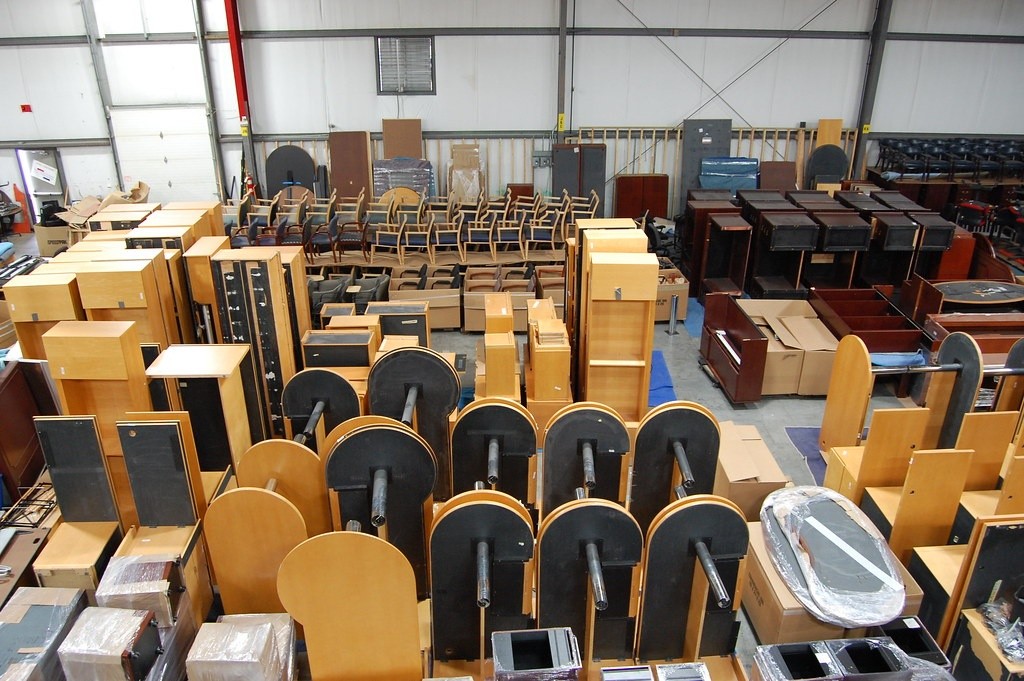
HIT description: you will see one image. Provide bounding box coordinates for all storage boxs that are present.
[0,552,299,681]
[712,420,925,645]
[738,296,841,397]
[654,256,689,320]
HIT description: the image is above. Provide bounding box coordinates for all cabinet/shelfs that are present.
[2,200,226,453]
[808,285,923,351]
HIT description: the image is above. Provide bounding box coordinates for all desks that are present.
[681,188,955,305]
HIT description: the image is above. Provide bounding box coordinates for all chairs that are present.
[221,186,600,263]
[874,139,1024,182]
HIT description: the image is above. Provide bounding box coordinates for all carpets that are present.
[784,425,869,487]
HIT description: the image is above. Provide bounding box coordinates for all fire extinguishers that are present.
[247,172,254,197]
